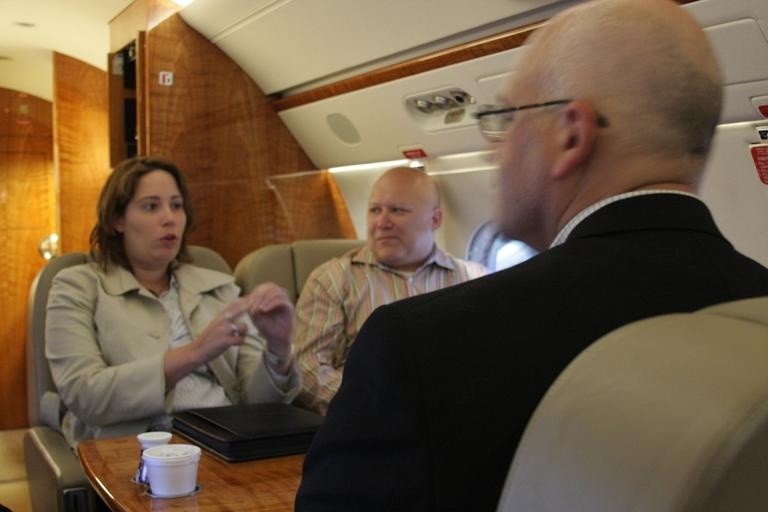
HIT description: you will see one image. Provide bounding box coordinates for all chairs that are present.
[495,296,768,512]
[24,245,237,512]
[234,238,367,302]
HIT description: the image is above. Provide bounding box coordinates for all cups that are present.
[142,443,201,497]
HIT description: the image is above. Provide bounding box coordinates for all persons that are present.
[44,158,295,441]
[294,0,768,511]
[292,166,496,416]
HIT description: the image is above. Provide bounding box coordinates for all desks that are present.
[77,431,305,512]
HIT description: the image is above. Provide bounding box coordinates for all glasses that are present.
[473,98,609,141]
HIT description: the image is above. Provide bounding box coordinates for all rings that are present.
[224,311,233,320]
[231,324,237,334]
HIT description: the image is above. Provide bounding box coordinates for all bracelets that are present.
[264,350,293,366]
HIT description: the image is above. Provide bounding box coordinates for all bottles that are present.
[130,432,174,487]
